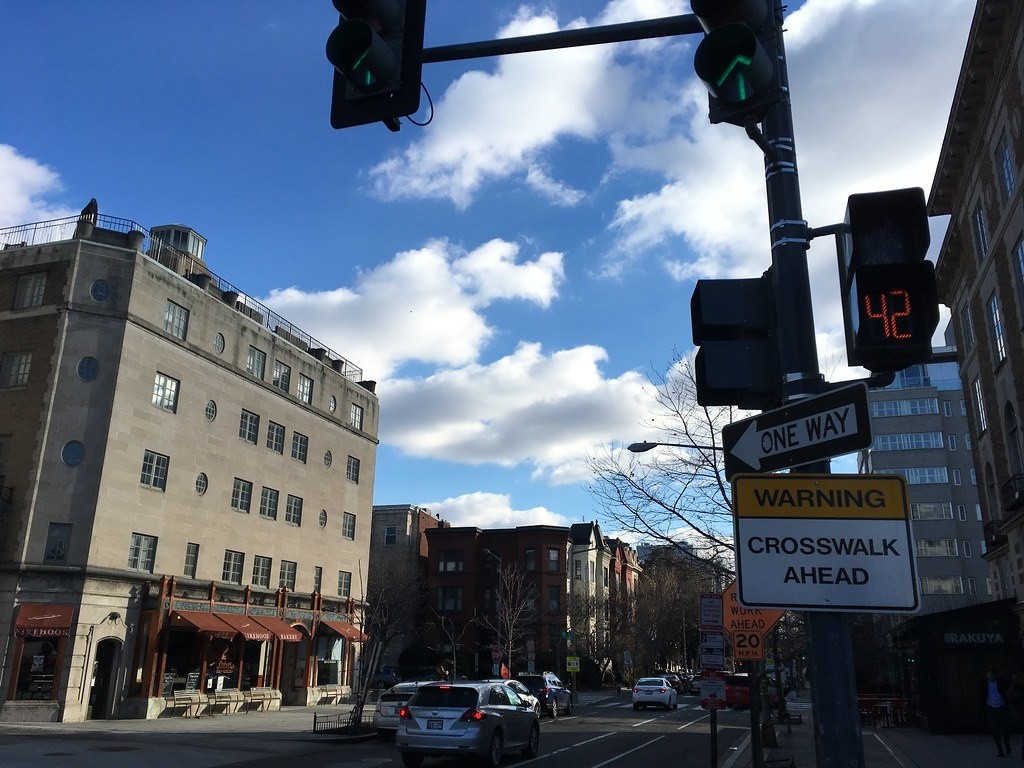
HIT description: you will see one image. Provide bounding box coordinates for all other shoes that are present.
[997,752,1005,757]
[1006,747,1012,754]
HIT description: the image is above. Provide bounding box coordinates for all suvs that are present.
[513,670,575,723]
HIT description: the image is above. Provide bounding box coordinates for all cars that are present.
[393,683,542,766]
[472,678,542,720]
[633,676,679,712]
[660,668,734,702]
[726,672,787,710]
[374,678,443,739]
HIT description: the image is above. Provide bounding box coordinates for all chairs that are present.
[858,699,877,728]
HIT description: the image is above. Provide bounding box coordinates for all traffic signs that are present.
[721,381,875,483]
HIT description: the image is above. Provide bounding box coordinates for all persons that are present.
[980,669,1014,758]
[436,665,451,681]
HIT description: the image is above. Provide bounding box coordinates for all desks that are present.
[873,704,898,728]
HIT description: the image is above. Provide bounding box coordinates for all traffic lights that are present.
[323,0,426,132]
[689,274,788,415]
[689,0,783,130]
[834,187,942,375]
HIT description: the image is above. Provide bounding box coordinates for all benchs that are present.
[211,688,249,714]
[248,686,282,713]
[319,683,352,705]
[170,689,212,719]
